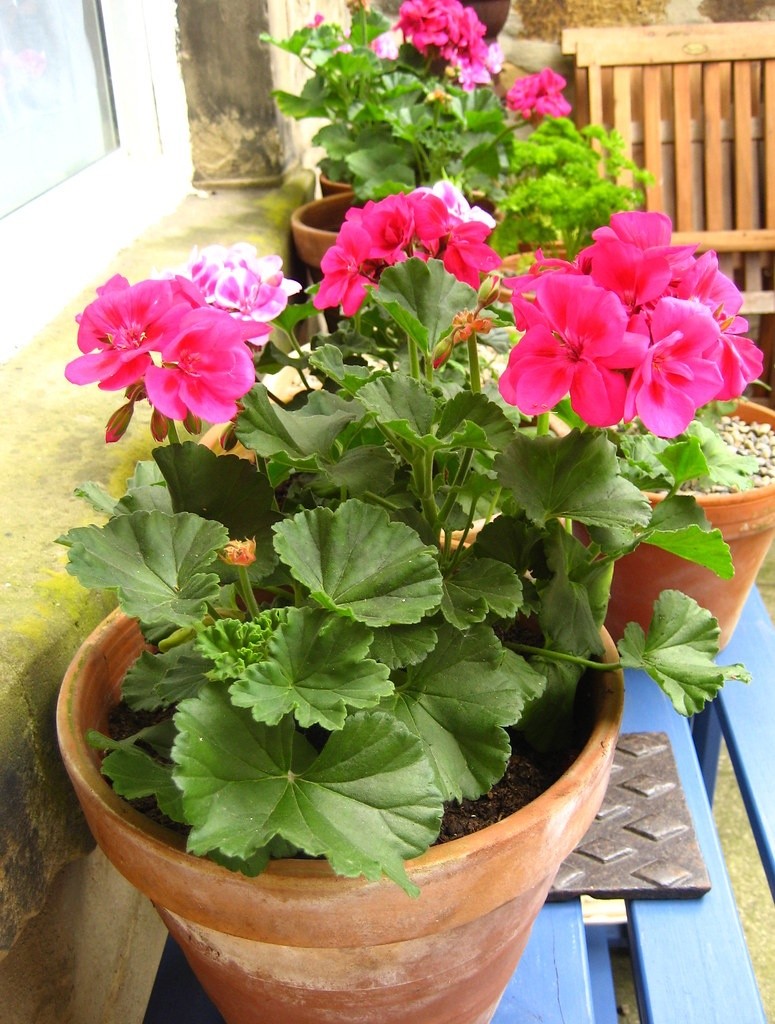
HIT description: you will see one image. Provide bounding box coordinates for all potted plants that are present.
[572,381,775,654]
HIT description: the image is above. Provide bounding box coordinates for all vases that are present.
[54,606,623,1024]
[290,192,505,336]
[197,387,571,546]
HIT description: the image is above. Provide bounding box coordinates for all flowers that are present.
[258,0,573,203]
[181,179,553,521]
[60,188,766,900]
